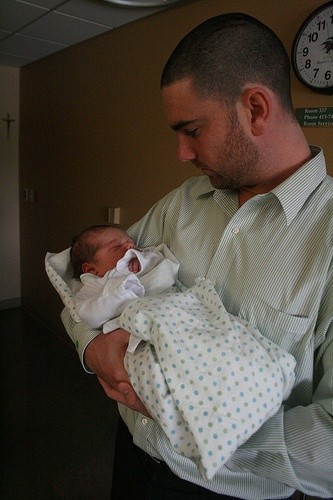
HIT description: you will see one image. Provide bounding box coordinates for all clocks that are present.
[291,0,333,96]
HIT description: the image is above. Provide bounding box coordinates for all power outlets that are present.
[108,207,121,224]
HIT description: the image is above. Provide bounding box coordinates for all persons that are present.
[69,225,182,332]
[58,12,332,500]
